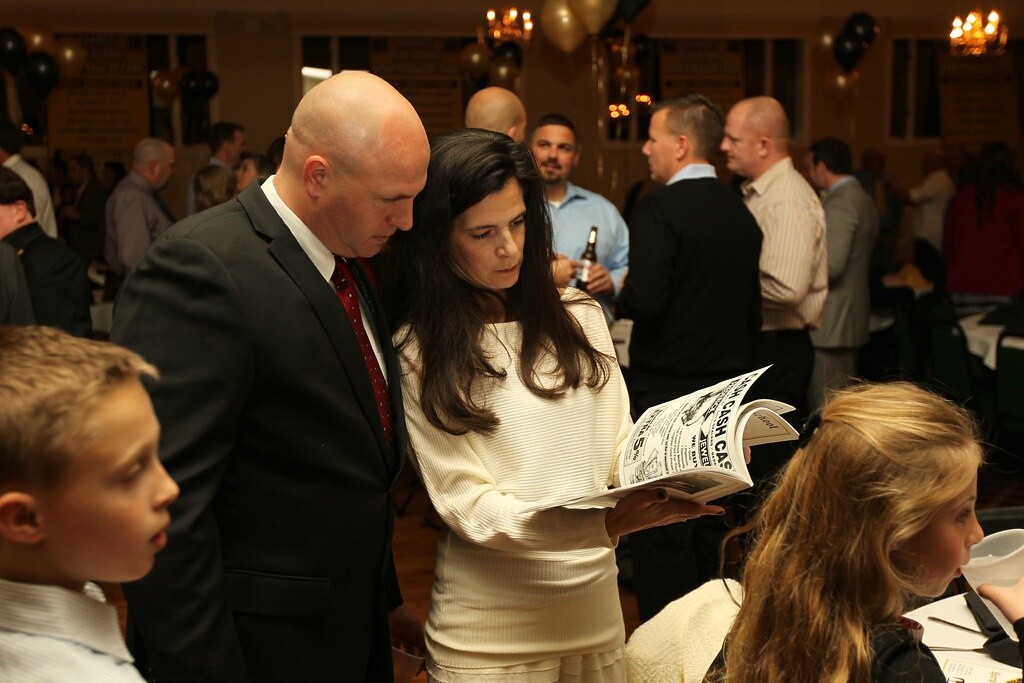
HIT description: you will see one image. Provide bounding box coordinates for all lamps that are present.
[481,5,535,59]
[951,6,1008,60]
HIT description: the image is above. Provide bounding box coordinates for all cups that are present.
[960,528,1024,641]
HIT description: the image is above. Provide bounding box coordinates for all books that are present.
[520,363,801,537]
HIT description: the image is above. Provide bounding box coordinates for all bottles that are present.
[575,226,598,294]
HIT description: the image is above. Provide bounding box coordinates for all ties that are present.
[330,257,397,462]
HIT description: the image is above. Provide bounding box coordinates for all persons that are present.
[612,92,1024,625]
[394,128,750,682]
[103,68,628,682]
[699,379,1024,682]
[1,122,103,339]
[0,324,181,683]
